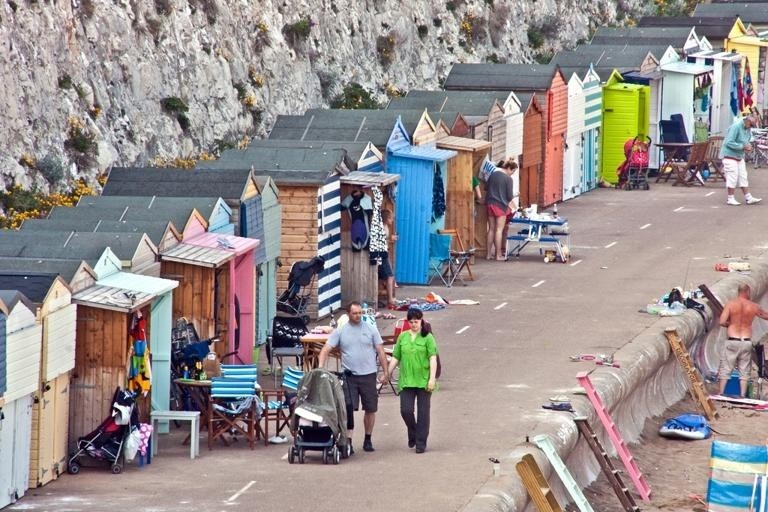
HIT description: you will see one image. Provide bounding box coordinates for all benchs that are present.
[503,212,571,263]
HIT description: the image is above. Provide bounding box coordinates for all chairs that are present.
[438,229,475,282]
[207,378,256,450]
[376,319,411,396]
[653,112,767,188]
[265,315,312,369]
[220,365,261,440]
[261,366,306,446]
[430,234,470,289]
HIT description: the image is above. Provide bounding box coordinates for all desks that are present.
[173,378,235,445]
[301,331,342,372]
[150,411,200,459]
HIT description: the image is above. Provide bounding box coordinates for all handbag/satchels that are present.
[124,426,140,464]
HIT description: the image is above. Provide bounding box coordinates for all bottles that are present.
[183,361,208,380]
[329,315,336,330]
[748,379,754,398]
[524,211,528,220]
[552,203,558,220]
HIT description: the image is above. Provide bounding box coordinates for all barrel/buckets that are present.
[725,372,740,395]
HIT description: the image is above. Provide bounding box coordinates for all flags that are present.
[744,56,755,107]
[729,61,740,116]
[738,59,745,113]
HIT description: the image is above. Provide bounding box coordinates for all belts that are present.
[728,338,753,342]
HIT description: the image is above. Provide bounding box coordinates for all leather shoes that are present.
[362,441,374,451]
[409,440,424,452]
[268,436,288,444]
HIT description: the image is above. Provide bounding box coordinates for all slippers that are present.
[542,393,572,411]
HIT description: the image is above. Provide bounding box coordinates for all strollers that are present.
[67,381,144,476]
[614,134,651,190]
[286,368,351,465]
[277,256,326,325]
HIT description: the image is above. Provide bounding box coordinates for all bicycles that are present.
[171,332,239,428]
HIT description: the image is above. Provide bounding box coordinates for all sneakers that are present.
[746,198,762,204]
[727,199,741,206]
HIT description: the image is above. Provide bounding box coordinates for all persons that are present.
[379,306,439,454]
[718,115,762,206]
[317,300,389,452]
[472,173,482,232]
[376,208,401,306]
[715,283,768,399]
[485,155,521,261]
